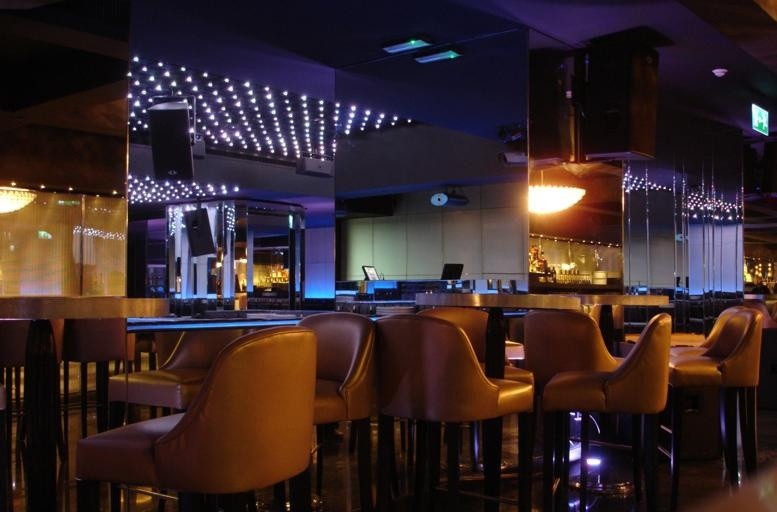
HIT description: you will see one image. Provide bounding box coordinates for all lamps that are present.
[528,168,587,215]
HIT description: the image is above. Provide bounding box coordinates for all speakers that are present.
[148,101,194,179]
[184,209,216,257]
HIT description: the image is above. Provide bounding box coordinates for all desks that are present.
[2,294,172,512]
[415,293,671,508]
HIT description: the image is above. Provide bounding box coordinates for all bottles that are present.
[537,266,556,283]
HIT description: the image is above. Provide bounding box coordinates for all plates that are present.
[376,306,415,317]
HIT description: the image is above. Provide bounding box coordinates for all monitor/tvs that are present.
[362,266,380,281]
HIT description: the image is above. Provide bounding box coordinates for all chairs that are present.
[77,325,318,512]
[415,306,533,384]
[543,311,672,511]
[669,295,777,512]
[523,306,616,512]
[110,327,258,511]
[62,317,136,464]
[375,313,535,510]
[273,311,374,511]
[2,318,64,484]
